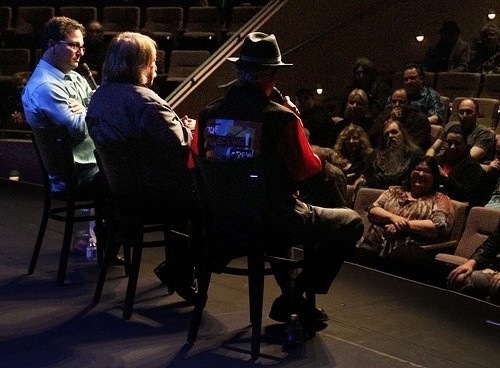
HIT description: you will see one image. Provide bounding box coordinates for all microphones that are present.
[79,62,98,89]
[270,87,285,105]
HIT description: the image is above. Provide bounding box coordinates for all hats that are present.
[227,31,293,66]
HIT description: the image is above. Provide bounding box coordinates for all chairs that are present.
[93,127,169,321]
[24,122,95,285]
[356,72,500,264]
[0,6,260,82]
[185,110,315,362]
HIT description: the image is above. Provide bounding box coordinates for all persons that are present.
[468,22,500,74]
[20,16,130,269]
[292,55,500,317]
[185,31,363,323]
[421,20,472,75]
[85,31,199,305]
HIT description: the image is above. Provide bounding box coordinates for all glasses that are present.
[57,40,86,55]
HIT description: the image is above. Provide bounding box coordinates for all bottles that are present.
[86,238,97,261]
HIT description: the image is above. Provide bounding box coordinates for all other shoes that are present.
[72,238,97,252]
[110,255,125,265]
[269,295,307,322]
[154,262,167,281]
[298,305,329,324]
[167,277,209,307]
